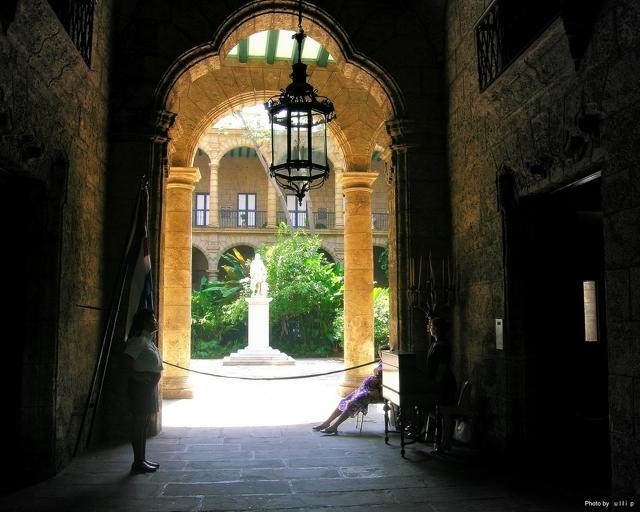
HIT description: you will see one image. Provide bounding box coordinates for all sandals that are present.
[311,426,339,435]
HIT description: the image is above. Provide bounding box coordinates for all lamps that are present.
[263,1,336,206]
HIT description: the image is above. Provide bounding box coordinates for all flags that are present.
[122,186,156,342]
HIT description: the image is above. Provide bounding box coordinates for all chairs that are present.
[426,381,473,449]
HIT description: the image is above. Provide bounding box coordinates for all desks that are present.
[380,385,444,458]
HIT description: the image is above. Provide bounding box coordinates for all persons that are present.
[312,343,393,436]
[426,315,462,456]
[249,252,269,298]
[122,307,163,473]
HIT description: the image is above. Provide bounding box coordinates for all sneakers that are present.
[132,460,161,473]
